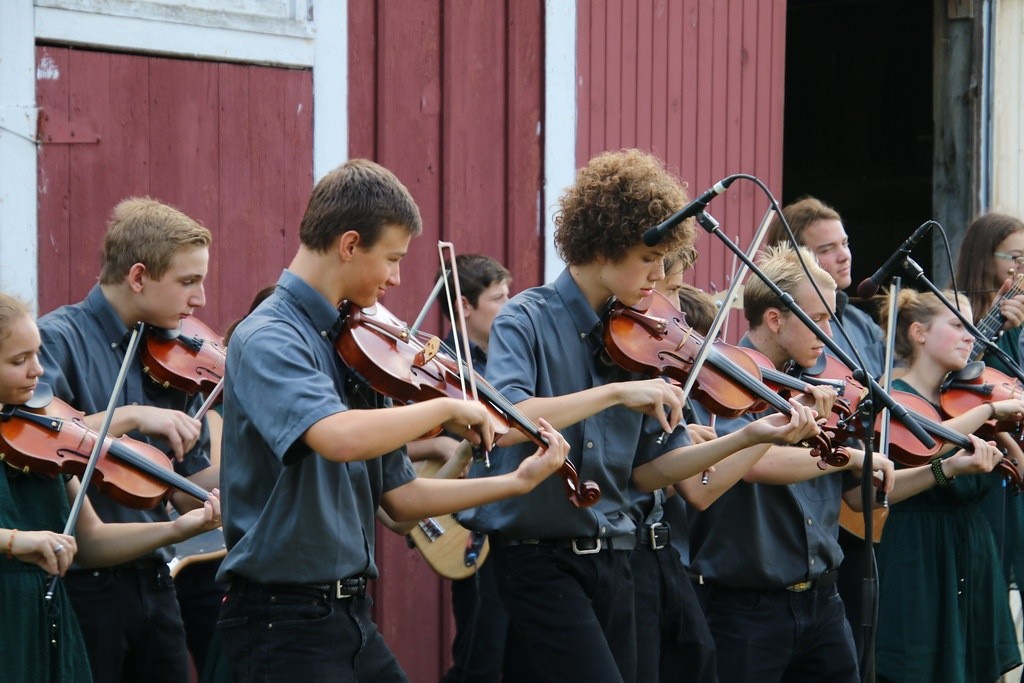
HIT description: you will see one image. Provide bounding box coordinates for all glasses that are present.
[994,252,1024,265]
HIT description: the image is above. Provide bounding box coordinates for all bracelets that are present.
[931,457,956,491]
[7,528,17,560]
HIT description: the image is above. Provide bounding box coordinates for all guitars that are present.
[399,457,490,581]
[836,256,1024,545]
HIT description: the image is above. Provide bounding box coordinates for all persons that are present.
[38,193,513,683]
[0,294,222,683]
[201,157,570,683]
[652,189,1024,683]
[471,149,821,683]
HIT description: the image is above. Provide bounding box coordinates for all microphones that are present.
[857,220,933,299]
[642,172,736,248]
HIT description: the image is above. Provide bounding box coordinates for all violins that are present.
[941,361,1023,442]
[140,312,228,402]
[335,298,600,507]
[600,289,850,472]
[651,336,864,438]
[776,349,1023,493]
[0,382,211,511]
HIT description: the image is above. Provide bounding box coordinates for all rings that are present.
[54,545,64,554]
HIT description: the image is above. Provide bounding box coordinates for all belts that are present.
[637,520,672,551]
[306,573,368,596]
[492,534,609,555]
[701,567,838,603]
